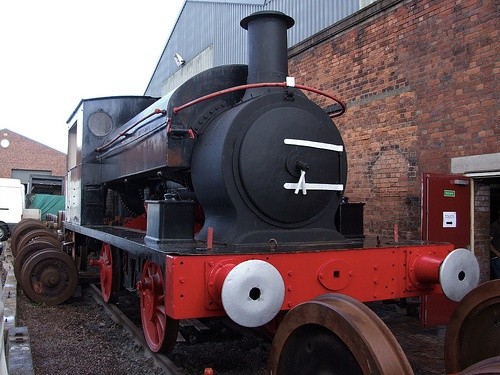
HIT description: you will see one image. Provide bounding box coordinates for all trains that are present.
[8,10,478,375]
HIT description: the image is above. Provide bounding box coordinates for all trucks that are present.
[0,177,26,241]
